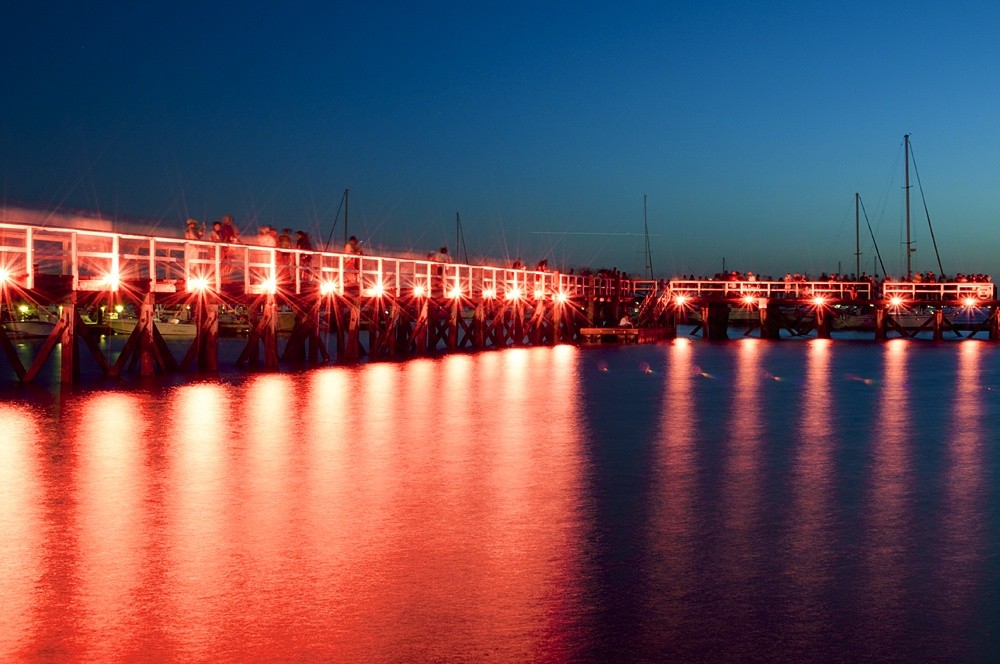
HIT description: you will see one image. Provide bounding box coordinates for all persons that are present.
[680,272,993,301]
[344,236,364,283]
[435,245,451,285]
[512,258,526,277]
[185,214,314,290]
[535,259,632,299]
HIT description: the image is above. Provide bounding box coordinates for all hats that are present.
[186,219,197,224]
